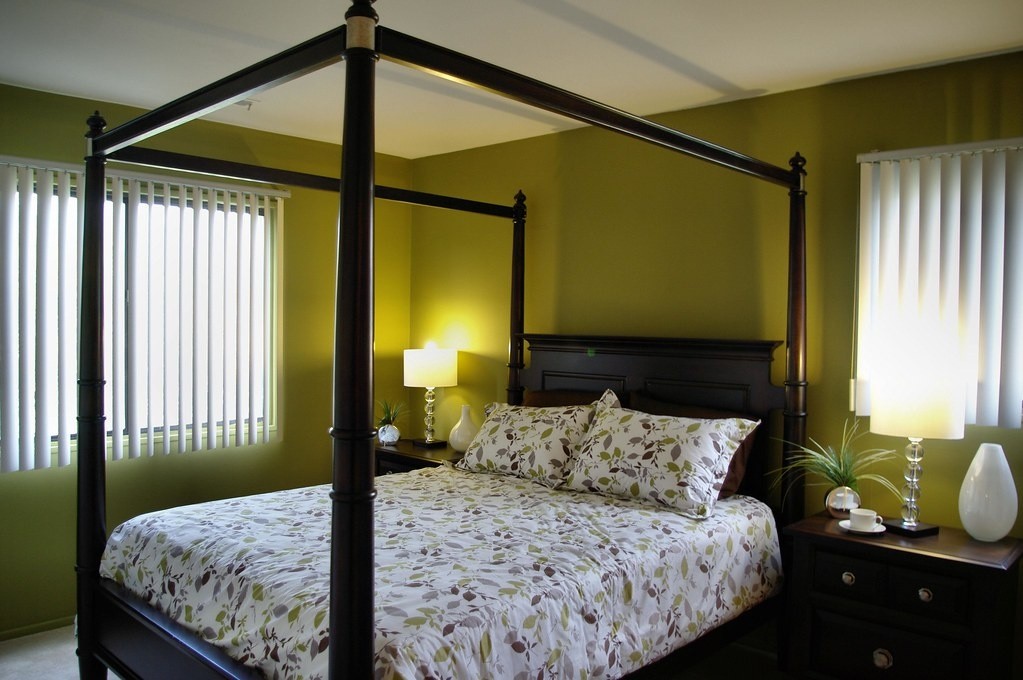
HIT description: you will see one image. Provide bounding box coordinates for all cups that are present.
[850,508,883,530]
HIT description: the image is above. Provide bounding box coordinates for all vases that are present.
[449,404,477,452]
[958,443,1018,545]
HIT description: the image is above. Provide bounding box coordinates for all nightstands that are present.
[782,508,1023,680]
[374,439,465,478]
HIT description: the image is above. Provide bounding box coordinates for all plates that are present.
[838,519,887,533]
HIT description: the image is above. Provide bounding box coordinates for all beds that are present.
[74,1,806,680]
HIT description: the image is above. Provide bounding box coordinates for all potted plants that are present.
[375,399,410,446]
[763,417,921,522]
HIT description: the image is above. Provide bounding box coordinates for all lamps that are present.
[866,309,965,539]
[403,349,457,449]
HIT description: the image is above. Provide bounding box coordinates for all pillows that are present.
[443,401,597,489]
[552,400,762,517]
